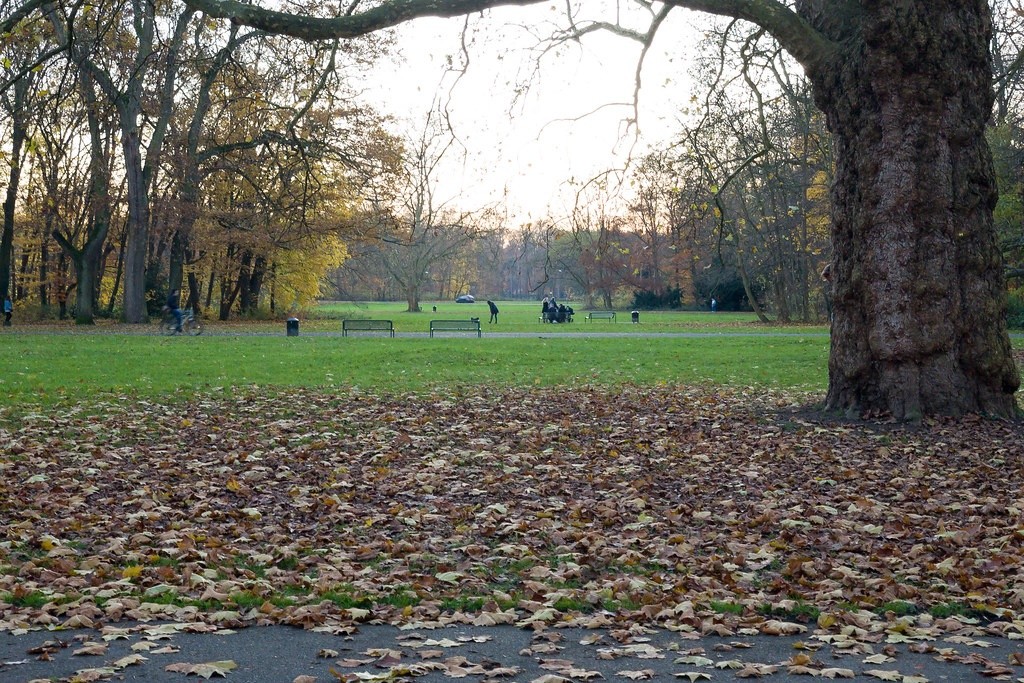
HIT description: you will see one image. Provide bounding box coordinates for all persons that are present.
[165,290,185,332]
[541,295,574,324]
[487,300,499,324]
[4,295,13,321]
[711,297,721,313]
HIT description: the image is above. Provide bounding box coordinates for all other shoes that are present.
[176,326,182,332]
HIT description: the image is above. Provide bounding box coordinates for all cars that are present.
[456,295,475,303]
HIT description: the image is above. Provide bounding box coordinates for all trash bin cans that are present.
[632,311,639,323]
[287,318,299,337]
[433,306,436,311]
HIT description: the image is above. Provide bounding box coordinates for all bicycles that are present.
[158,308,204,338]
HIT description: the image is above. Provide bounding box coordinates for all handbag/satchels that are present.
[553,320,557,324]
[162,305,172,314]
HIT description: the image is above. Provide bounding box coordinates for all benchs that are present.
[342,320,395,338]
[430,320,481,338]
[539,312,573,324]
[585,312,616,324]
